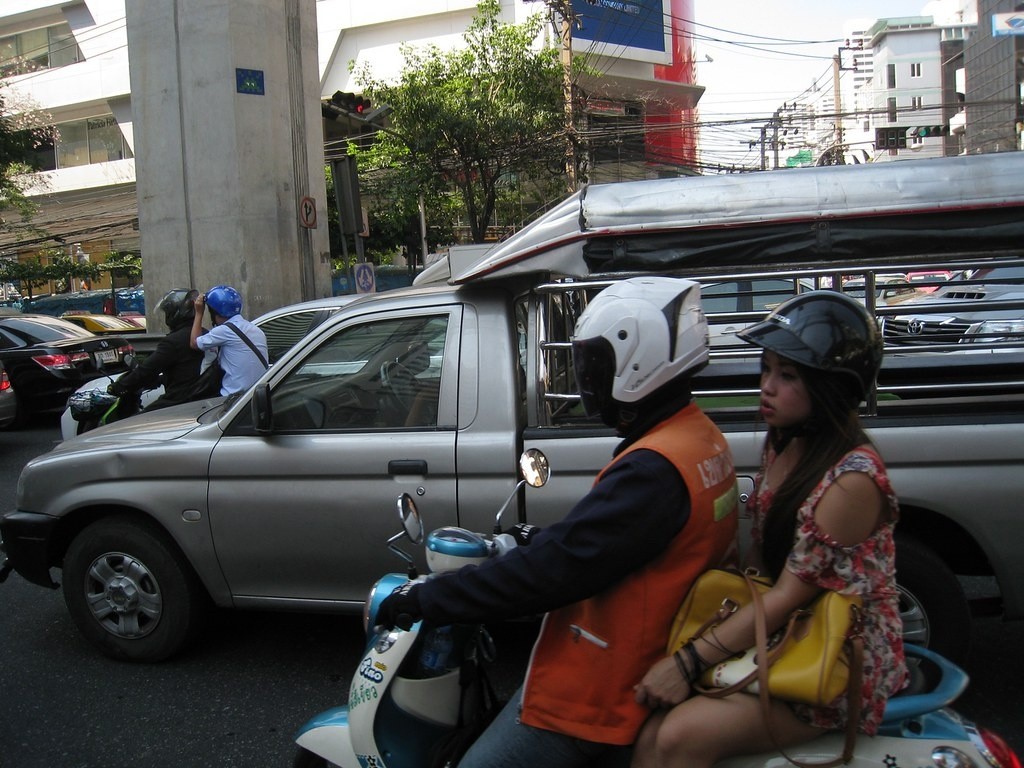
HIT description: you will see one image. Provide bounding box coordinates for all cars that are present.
[61,315,146,335]
[698,279,815,346]
[843,271,1024,357]
[59,294,448,439]
[0,315,139,431]
[104,284,145,316]
[0,359,17,430]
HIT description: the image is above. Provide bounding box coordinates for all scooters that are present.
[293,448,1021,768]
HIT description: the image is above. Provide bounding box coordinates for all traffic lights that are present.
[917,125,950,137]
[875,127,910,149]
[333,91,371,115]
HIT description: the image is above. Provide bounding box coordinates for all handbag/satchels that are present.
[666,568,855,707]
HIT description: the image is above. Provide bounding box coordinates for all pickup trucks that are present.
[0,152,1024,698]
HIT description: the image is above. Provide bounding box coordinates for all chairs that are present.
[406,386,439,425]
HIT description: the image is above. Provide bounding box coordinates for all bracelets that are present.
[673,626,747,686]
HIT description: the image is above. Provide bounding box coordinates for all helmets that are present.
[572,276,710,403]
[734,290,884,397]
[161,289,202,331]
[203,285,242,317]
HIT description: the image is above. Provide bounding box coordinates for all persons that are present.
[631,291,911,768]
[372,276,739,768]
[190,286,268,398]
[107,288,218,415]
[404,383,439,427]
[20,288,30,304]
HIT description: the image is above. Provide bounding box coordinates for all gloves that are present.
[375,583,423,633]
[507,523,539,548]
